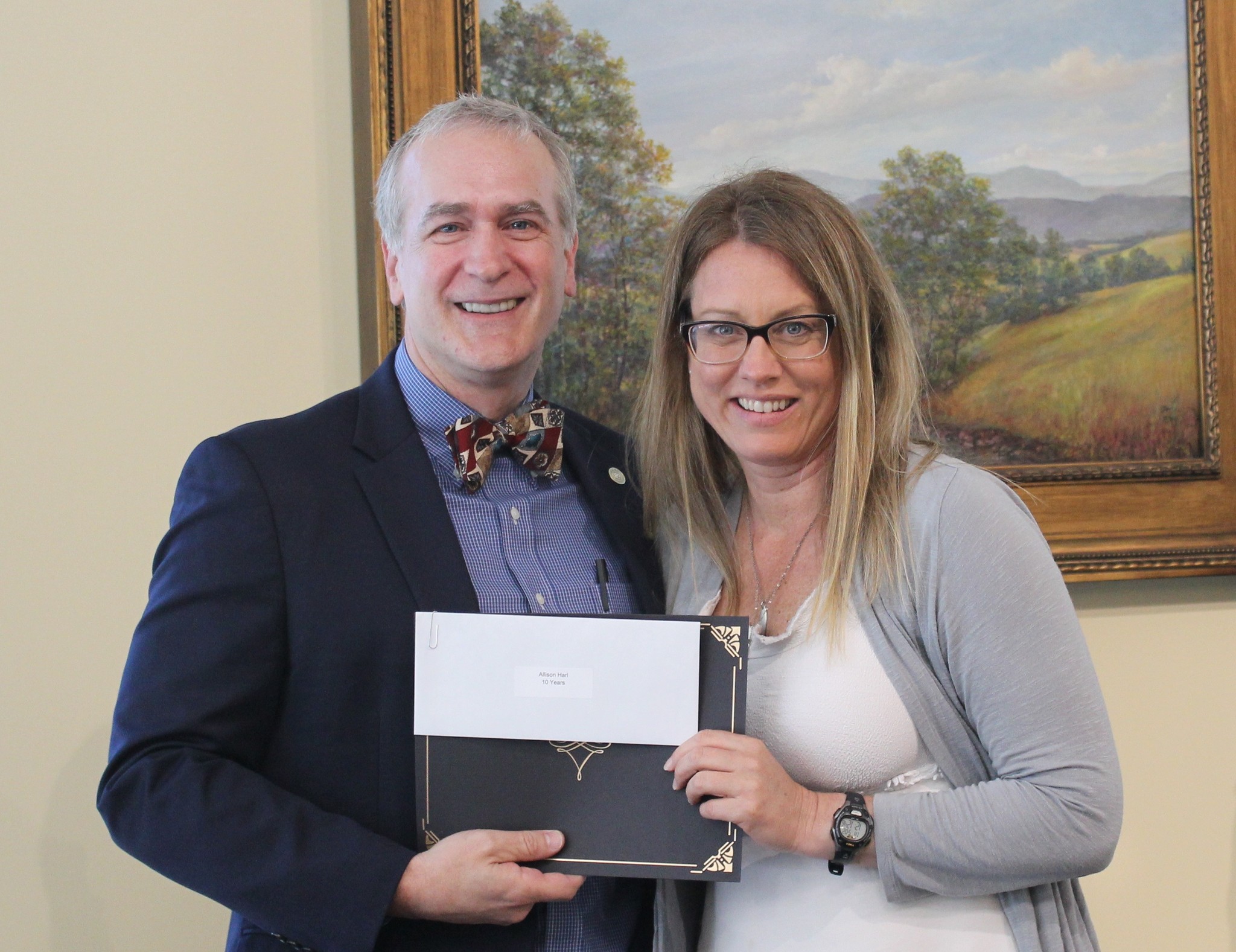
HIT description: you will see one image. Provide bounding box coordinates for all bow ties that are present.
[444,398,565,495]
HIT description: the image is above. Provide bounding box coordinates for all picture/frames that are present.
[365,0,1236,583]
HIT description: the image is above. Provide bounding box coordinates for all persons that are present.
[620,162,1127,952]
[93,91,705,952]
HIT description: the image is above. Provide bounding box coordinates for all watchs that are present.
[827,791,875,876]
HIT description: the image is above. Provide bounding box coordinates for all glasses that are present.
[679,314,837,365]
